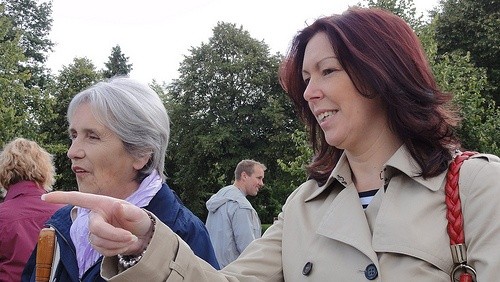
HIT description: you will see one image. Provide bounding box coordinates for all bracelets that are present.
[118,215,156,267]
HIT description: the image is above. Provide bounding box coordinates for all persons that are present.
[21,79,220,282]
[0,138,64,282]
[206,160,267,270]
[41,8,500,282]
